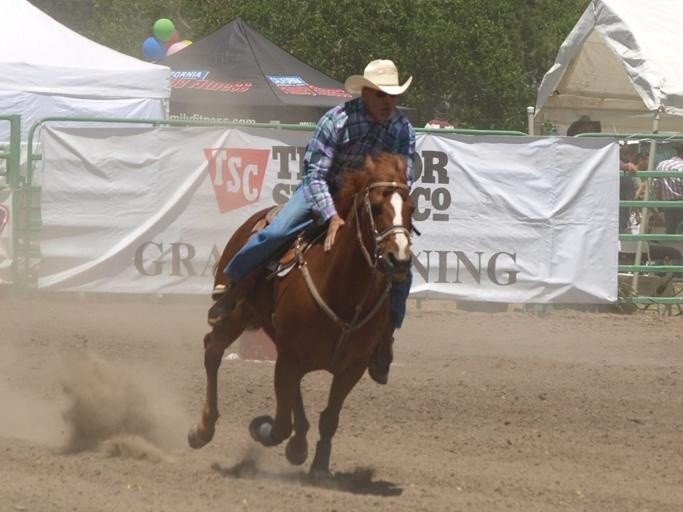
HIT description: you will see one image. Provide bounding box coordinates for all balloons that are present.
[141,18,192,63]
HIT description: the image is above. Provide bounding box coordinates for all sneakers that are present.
[221,283,245,316]
[369,337,394,384]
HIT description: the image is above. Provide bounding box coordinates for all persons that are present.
[207,60,415,384]
[620,144,683,277]
[424,101,455,130]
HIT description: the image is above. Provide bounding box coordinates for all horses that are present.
[185,151,413,476]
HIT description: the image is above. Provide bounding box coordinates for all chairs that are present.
[618,240,682,316]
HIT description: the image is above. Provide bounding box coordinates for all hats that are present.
[344,60,413,96]
[568,115,601,136]
